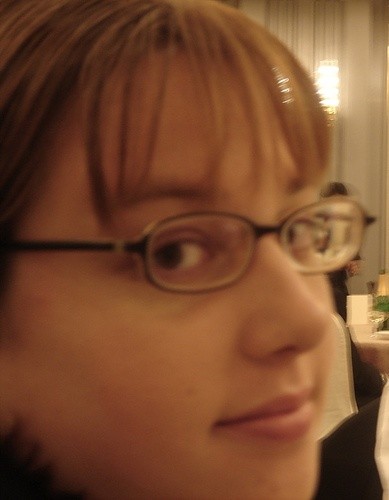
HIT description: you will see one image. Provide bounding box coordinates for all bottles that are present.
[372,269,389,330]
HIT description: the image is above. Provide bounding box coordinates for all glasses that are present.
[1,195,368,293]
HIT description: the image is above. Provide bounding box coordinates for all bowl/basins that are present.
[375,331,389,340]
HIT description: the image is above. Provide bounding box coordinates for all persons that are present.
[311,377,388,500]
[327,288,385,406]
[316,182,381,325]
[0,1,341,500]
